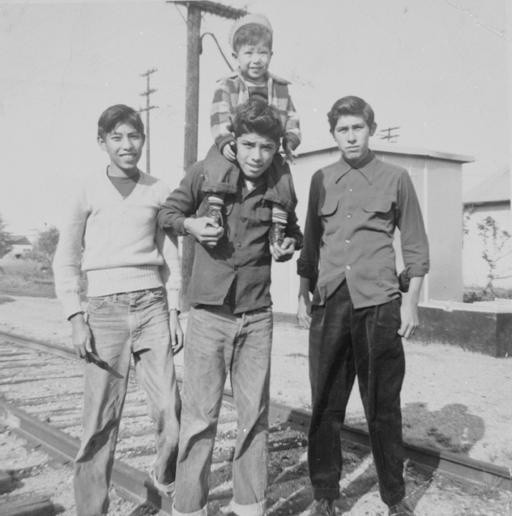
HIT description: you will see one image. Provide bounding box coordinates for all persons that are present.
[294,93,432,516]
[209,11,303,165]
[50,102,184,515]
[155,99,304,515]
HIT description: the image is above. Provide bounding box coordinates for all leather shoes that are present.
[267,225,286,251]
[205,207,223,229]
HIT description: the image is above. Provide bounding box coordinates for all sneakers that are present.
[310,497,337,516]
[388,500,414,515]
